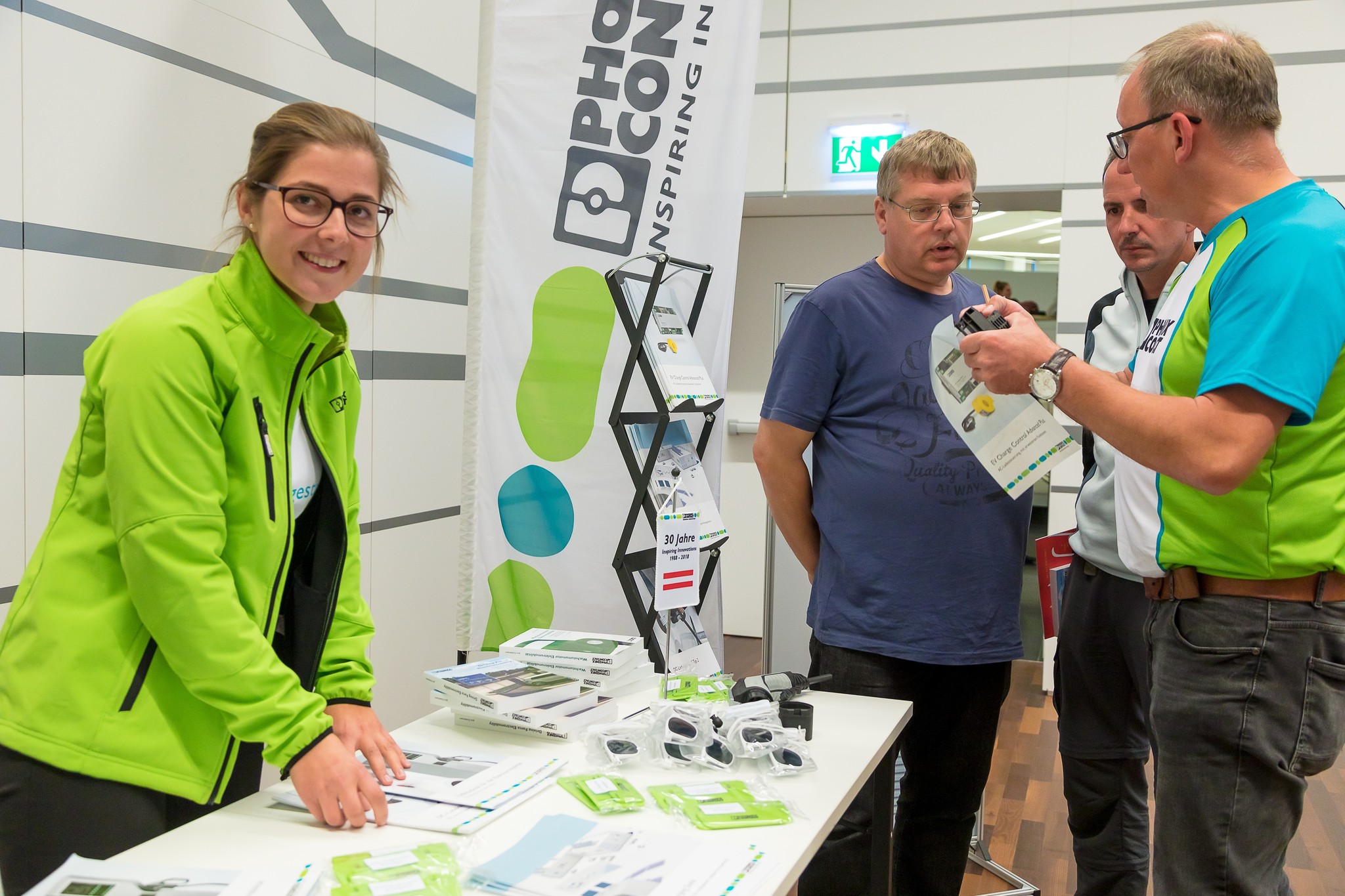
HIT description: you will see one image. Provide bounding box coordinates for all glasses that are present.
[250,180,394,238]
[597,706,804,776]
[882,195,982,222]
[1106,113,1202,159]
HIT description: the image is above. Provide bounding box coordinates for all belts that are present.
[1142,566,1345,602]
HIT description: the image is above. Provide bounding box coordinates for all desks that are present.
[103,671,915,896]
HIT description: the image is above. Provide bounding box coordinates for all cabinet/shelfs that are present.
[604,253,725,673]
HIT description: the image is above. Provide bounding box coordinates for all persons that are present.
[1052,133,1206,896]
[751,130,1033,896]
[0,101,411,896]
[993,280,1045,316]
[950,24,1345,896]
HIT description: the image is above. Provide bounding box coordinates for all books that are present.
[262,627,660,835]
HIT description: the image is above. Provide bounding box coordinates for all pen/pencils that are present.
[981,285,990,304]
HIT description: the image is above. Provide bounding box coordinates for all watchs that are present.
[1028,349,1077,402]
